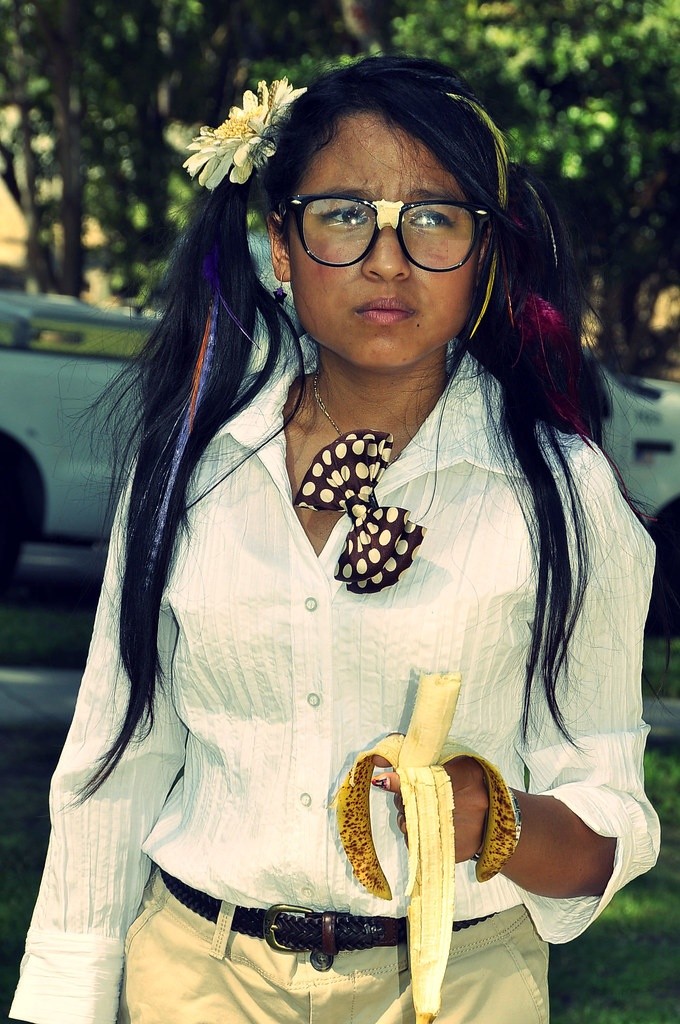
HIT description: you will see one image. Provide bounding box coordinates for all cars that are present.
[0,289,158,595]
[597,363,680,636]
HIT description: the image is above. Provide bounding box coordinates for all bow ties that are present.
[291,428,427,594]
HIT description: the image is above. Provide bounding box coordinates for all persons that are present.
[8,53,663,1024]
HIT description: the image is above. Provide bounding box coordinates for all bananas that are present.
[335,672,518,1024]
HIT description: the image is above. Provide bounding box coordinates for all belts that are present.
[159,869,501,957]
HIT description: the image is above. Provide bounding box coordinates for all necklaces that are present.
[309,359,347,437]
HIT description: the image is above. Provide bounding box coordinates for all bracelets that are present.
[474,782,523,864]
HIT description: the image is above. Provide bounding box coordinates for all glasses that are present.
[278,193,492,273]
